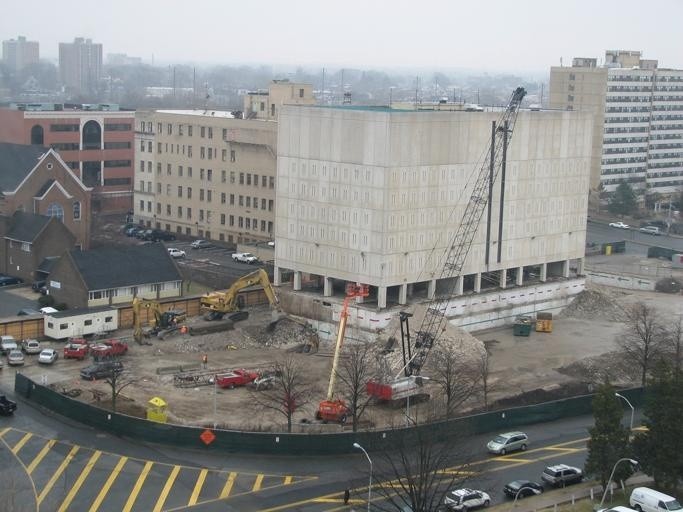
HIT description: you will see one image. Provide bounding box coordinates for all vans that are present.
[629,486,682,511]
[638,225,660,236]
[486,431,529,455]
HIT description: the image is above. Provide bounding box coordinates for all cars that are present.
[115,222,260,267]
[608,221,629,230]
[502,479,543,499]
[0,275,128,417]
[597,505,640,512]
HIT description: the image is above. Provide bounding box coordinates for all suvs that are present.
[443,487,490,511]
[540,463,582,488]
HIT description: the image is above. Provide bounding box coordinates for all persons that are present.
[203,354,207,369]
[181,325,186,338]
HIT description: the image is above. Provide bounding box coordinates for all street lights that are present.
[509,485,540,512]
[598,457,638,509]
[352,442,372,511]
[615,394,634,444]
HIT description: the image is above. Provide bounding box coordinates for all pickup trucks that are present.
[216,367,261,391]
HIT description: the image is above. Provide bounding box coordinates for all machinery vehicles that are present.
[314,281,373,422]
[185,267,286,336]
[130,288,184,347]
[367,86,527,415]
